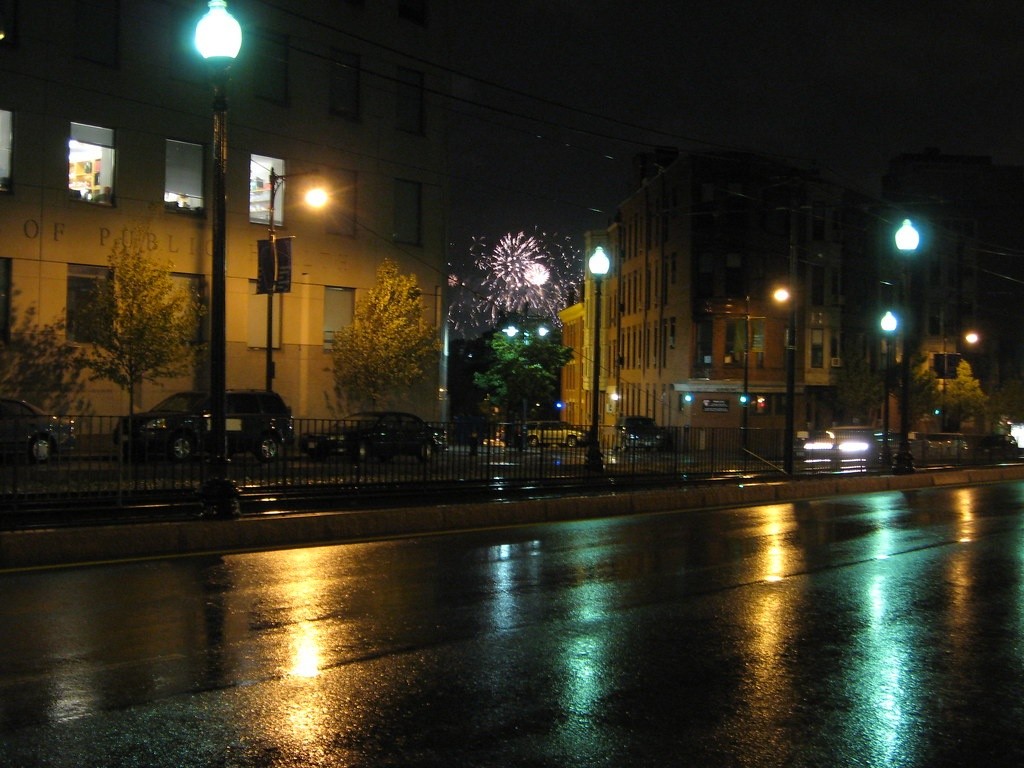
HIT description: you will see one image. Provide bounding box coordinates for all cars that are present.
[0,394,77,464]
[805,425,1019,466]
[299,411,448,465]
[614,416,668,453]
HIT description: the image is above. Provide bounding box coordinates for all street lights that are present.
[183,0,245,518]
[878,310,900,466]
[769,283,797,481]
[583,246,611,474]
[262,168,335,391]
[892,219,921,472]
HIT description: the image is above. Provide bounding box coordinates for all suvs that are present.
[496,420,590,448]
[111,389,295,465]
